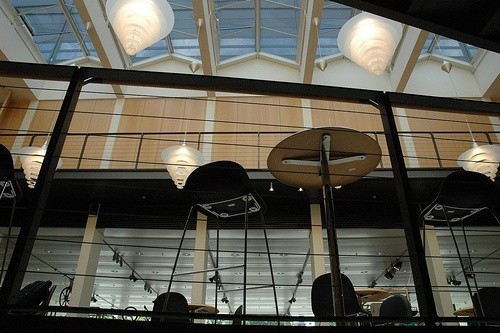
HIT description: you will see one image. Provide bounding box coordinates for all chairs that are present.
[473,287,500,325]
[232,305,243,325]
[379,295,413,320]
[150,292,190,323]
[312,273,364,326]
[9,285,57,316]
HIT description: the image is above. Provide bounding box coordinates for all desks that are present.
[188,304,219,323]
[453,306,475,318]
[355,288,388,309]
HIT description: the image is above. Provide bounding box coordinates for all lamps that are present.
[209,274,220,283]
[162,18,205,191]
[452,276,461,285]
[432,33,500,181]
[289,294,296,303]
[221,298,229,304]
[19,21,93,191]
[106,0,175,56]
[297,273,304,284]
[269,181,274,191]
[91,294,97,303]
[385,259,403,281]
[337,11,405,75]
[112,252,153,294]
